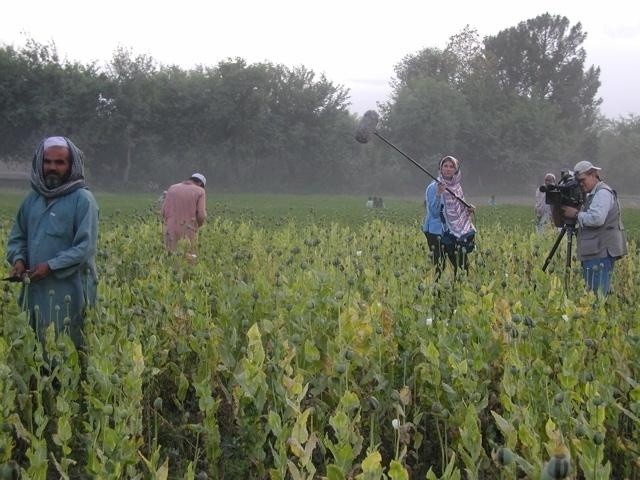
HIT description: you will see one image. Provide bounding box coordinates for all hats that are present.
[574,161,602,175]
[43,136,70,153]
[192,173,206,187]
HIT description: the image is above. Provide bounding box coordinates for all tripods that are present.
[535,227,586,299]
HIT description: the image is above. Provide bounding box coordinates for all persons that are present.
[488,194,496,206]
[160,172,206,269]
[563,160,628,304]
[5,135,99,466]
[367,196,385,208]
[423,156,477,299]
[536,174,563,236]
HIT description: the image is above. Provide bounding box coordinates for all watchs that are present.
[575,211,579,218]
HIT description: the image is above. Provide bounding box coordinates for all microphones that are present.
[356,110,379,143]
